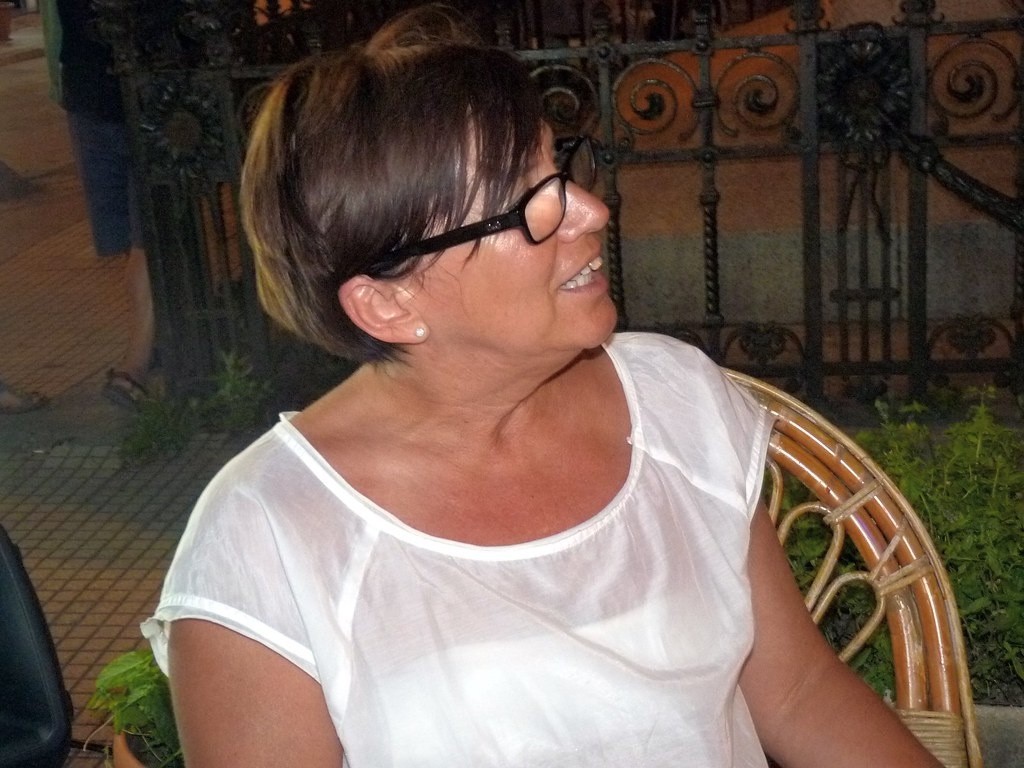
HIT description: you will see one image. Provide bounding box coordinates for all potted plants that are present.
[89,648,185,768]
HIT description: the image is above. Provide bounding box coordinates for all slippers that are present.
[104,362,149,414]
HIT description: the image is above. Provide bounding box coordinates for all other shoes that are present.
[0,381,51,415]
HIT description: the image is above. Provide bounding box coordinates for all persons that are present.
[138,10,946,768]
[0,1,178,415]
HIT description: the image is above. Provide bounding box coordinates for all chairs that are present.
[727,367,981,768]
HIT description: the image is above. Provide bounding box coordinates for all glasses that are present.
[390,134,599,265]
